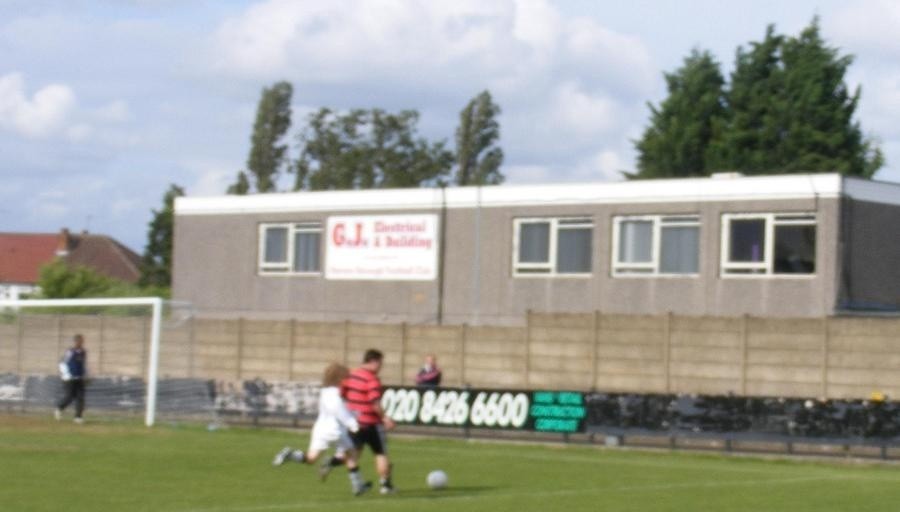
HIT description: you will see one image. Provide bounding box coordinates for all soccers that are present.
[426,470,447,489]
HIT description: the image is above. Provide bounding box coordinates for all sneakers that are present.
[74,418,83,424]
[320,456,332,481]
[55,409,63,420]
[353,480,372,496]
[380,482,393,495]
[274,448,293,466]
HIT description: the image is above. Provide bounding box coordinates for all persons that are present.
[321,347,401,494]
[415,354,441,385]
[52,333,87,422]
[274,364,372,498]
